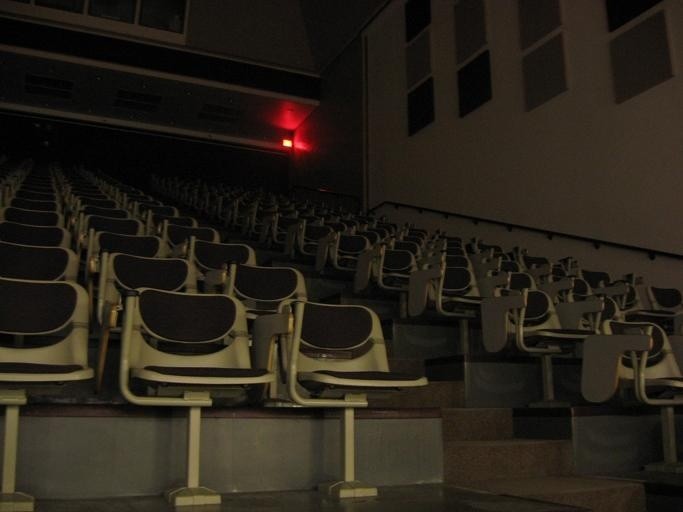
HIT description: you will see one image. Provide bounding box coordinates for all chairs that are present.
[0,276,102,511]
[111,287,278,506]
[579,318,683,475]
[137,173,682,409]
[1,158,308,408]
[279,296,428,498]
